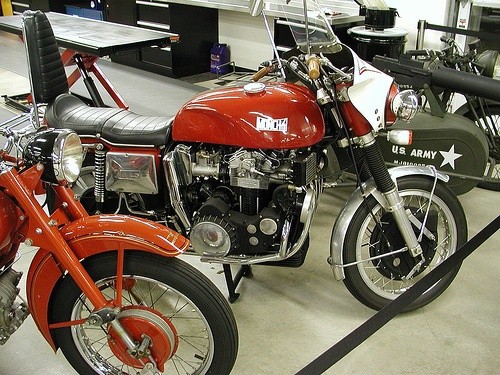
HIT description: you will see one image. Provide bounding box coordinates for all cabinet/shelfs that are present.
[273,13,366,74]
[0,0,219,79]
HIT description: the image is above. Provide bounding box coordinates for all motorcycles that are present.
[0,95,239,375]
[0,1,468,314]
[314,20,500,191]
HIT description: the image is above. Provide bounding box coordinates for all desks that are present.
[0,11,180,109]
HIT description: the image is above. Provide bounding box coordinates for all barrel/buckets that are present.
[211,43,230,73]
